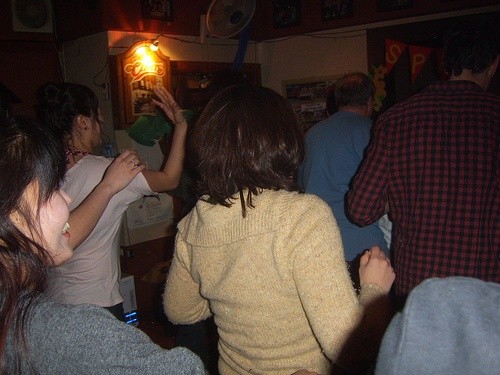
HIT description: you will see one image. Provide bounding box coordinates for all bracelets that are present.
[176,118,189,127]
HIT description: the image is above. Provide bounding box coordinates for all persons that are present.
[297,72,394,296]
[326,90,338,117]
[164,82,396,375]
[0,99,323,374]
[346,27,500,324]
[35,83,188,323]
[375,276,500,374]
[0,85,145,268]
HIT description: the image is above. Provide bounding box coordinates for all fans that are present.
[200,0,257,61]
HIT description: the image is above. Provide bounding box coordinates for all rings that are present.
[131,159,136,169]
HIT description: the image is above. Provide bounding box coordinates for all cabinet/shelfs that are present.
[170,61,261,109]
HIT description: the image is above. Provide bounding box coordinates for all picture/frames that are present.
[270,0,356,29]
[142,0,175,22]
